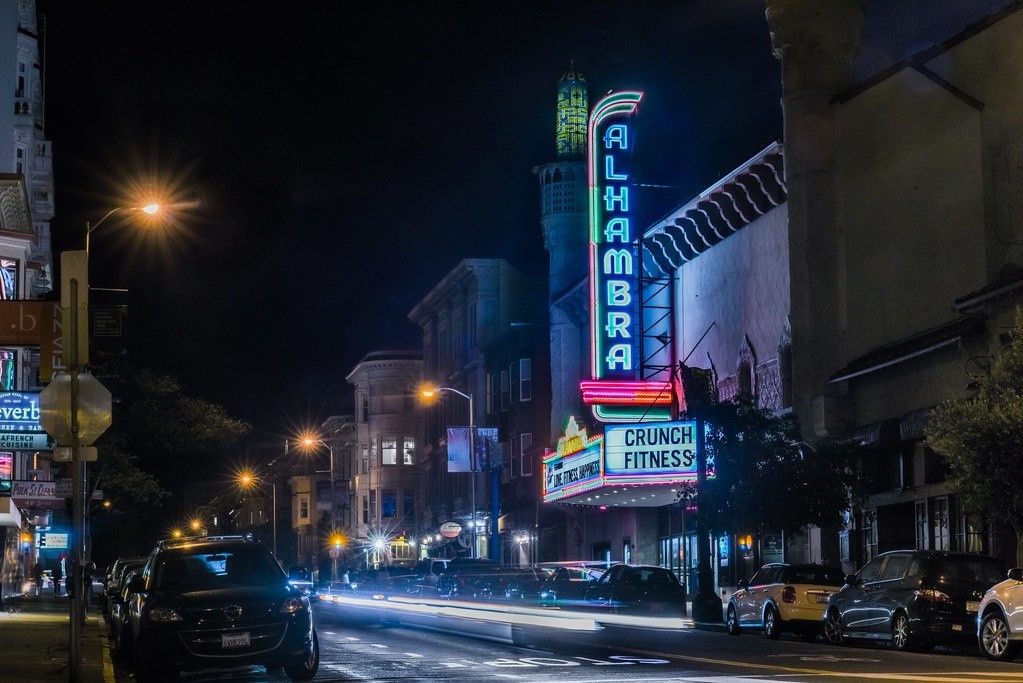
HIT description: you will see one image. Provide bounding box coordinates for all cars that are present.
[351,557,687,620]
[977,567,1023,661]
[820,549,1007,652]
[101,535,320,683]
[725,550,844,640]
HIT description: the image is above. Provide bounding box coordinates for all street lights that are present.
[240,476,277,559]
[424,386,477,559]
[82,201,160,269]
[306,438,337,532]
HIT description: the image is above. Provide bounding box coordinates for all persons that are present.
[51,564,62,594]
[32,558,43,596]
